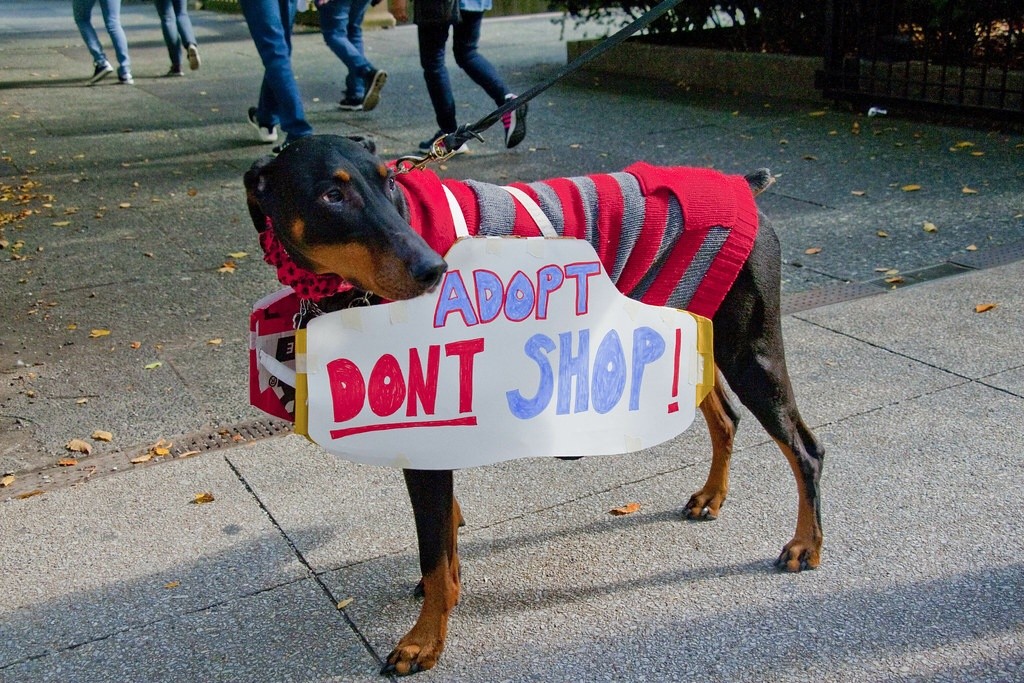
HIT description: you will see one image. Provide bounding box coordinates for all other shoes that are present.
[89,63,114,83]
[168,65,183,77]
[119,75,134,85]
[187,44,200,70]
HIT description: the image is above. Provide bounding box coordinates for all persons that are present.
[154,0,200,77]
[73,0,134,85]
[237,0,314,156]
[314,0,386,111]
[413,0,528,154]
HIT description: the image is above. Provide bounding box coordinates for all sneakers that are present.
[502,96,528,148]
[247,106,277,142]
[361,70,387,112]
[419,131,468,155]
[339,95,363,111]
[272,136,296,156]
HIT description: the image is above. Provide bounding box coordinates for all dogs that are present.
[243,134,825,678]
